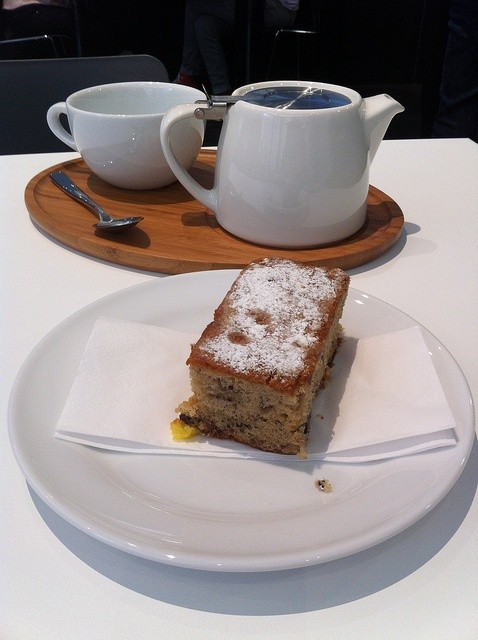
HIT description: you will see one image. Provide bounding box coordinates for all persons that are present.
[2,0,111,54]
[168,0,300,92]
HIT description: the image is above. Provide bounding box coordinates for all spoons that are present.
[48,169,144,233]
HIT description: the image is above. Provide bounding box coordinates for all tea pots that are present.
[159,80,406,250]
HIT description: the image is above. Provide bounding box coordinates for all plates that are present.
[4,268,477,575]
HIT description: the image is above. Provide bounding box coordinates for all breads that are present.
[170,256,351,457]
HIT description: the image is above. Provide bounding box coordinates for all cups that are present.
[46,81,209,192]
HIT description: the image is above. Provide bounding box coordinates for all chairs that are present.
[1,54,171,153]
